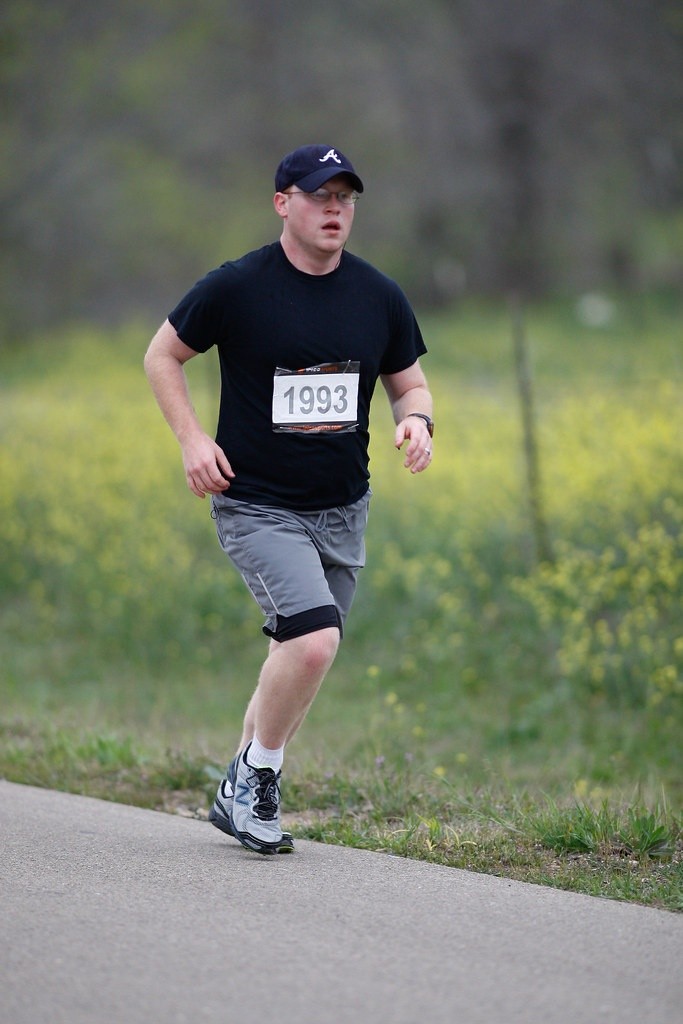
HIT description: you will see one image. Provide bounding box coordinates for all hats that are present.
[275,144,363,193]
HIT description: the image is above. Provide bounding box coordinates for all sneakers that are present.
[227,740,282,854]
[209,778,293,852]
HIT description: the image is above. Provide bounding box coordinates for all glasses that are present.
[281,188,359,205]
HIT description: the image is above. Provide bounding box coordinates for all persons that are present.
[144,145,433,855]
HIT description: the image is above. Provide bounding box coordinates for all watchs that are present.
[407,413,433,438]
